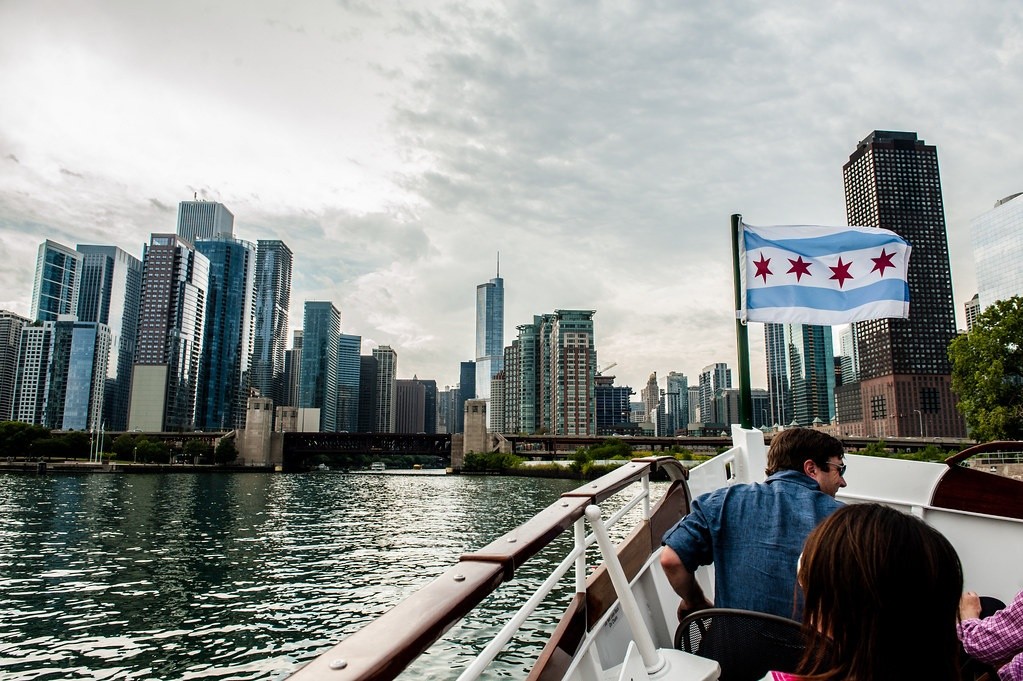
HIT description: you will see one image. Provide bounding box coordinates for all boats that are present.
[372,461,386,471]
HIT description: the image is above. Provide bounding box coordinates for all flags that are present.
[738,216,912,325]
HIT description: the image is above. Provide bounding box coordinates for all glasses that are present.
[825,461,846,477]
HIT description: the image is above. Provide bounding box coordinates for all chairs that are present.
[674,607,831,680]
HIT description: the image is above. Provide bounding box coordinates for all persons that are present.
[757,502,974,681]
[956,590,1023,680]
[660,425,847,681]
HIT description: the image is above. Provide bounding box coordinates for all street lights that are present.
[762,409,768,427]
[914,410,923,438]
